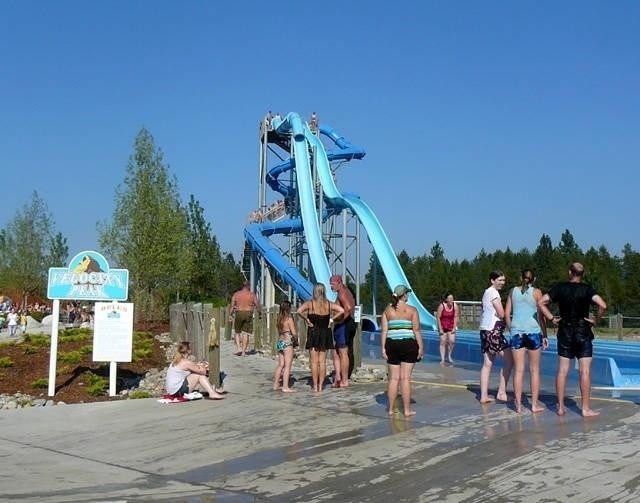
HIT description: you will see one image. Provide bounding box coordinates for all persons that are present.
[0,298,92,337]
[272,300,299,393]
[251,199,284,222]
[478,266,514,403]
[436,290,459,364]
[275,111,283,119]
[164,340,226,399]
[311,110,318,129]
[296,282,345,392]
[329,273,357,389]
[503,267,550,412]
[227,279,261,356]
[379,283,425,417]
[537,261,608,417]
[267,109,275,122]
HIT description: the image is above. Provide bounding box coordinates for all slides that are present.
[245,110,640,389]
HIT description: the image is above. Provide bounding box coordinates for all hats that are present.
[394,285,411,297]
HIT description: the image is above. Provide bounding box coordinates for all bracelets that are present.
[330,318,334,322]
[549,316,555,321]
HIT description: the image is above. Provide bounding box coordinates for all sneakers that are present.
[183,391,203,400]
